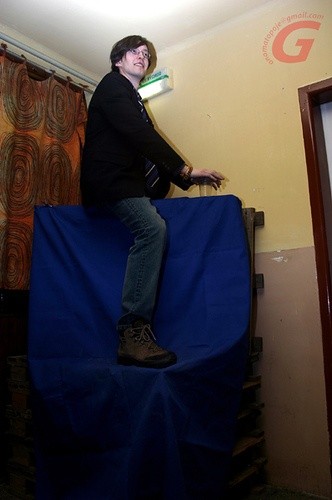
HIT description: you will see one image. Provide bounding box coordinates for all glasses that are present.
[128,48,152,59]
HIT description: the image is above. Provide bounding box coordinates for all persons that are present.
[80,35,227,367]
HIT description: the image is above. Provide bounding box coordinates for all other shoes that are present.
[116,321,177,367]
